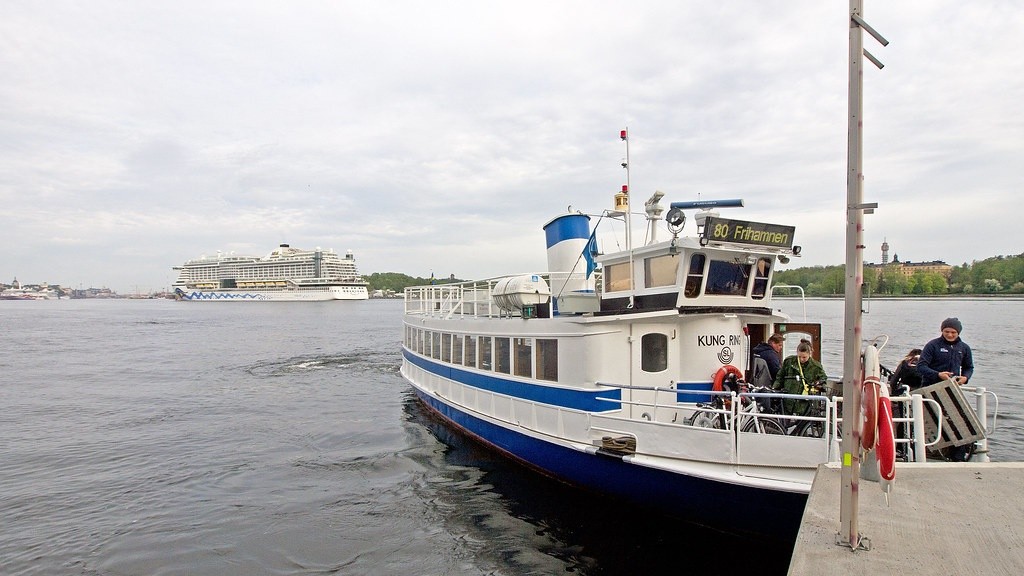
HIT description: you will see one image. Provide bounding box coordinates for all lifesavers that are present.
[714,365,746,405]
[860,346,897,480]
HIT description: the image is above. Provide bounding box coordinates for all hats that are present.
[941,317,962,335]
[770,333,785,343]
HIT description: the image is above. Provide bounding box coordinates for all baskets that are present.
[760,391,783,411]
[809,386,842,412]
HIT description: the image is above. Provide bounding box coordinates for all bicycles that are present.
[755,375,842,438]
[688,378,788,435]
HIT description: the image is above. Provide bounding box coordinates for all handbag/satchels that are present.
[801,384,819,400]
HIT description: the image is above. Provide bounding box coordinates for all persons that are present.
[890,349,923,459]
[916,317,973,462]
[772,339,828,437]
[751,332,786,414]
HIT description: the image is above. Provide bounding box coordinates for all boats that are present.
[38,287,60,300]
[398,126,998,540]
[60,295,69,299]
[0,285,38,300]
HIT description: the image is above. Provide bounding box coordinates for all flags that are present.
[582,232,598,281]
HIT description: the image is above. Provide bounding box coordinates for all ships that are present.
[172,244,370,301]
